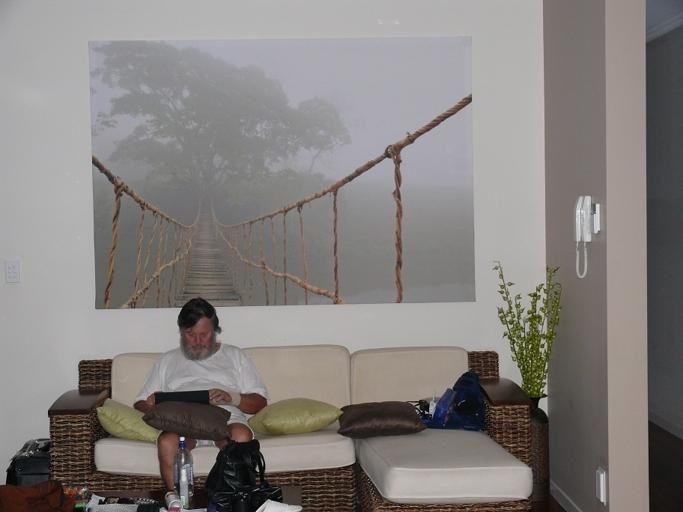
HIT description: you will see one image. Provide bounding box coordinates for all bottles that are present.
[164,436,196,512]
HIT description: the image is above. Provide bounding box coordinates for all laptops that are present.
[154,390,210,404]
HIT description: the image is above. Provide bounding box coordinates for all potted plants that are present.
[485,259,565,511]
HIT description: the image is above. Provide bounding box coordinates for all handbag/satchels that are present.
[206,439,266,512]
[429,372,483,432]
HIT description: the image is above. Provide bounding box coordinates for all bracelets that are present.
[227,391,241,406]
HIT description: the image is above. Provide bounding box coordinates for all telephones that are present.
[575,194,593,245]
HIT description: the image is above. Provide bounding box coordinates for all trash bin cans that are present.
[6,439,52,486]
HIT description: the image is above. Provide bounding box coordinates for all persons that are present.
[132,293,270,493]
